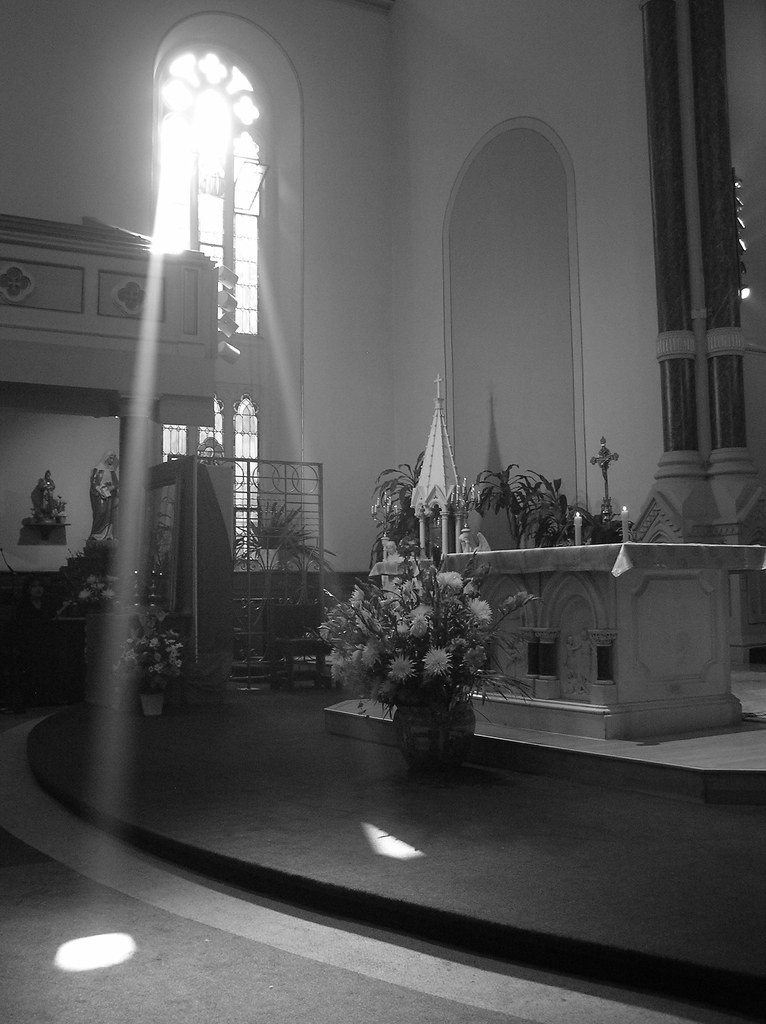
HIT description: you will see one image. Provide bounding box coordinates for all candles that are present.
[574,516,582,546]
[622,510,629,542]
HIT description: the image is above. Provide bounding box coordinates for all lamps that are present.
[450,478,481,552]
[371,493,399,560]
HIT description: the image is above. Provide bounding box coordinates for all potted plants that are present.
[233,500,338,576]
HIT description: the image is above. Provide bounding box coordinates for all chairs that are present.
[272,604,332,691]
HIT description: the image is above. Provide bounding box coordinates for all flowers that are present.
[66,548,125,613]
[111,608,198,694]
[318,542,543,726]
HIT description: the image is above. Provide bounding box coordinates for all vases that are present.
[394,702,476,786]
[141,694,164,716]
